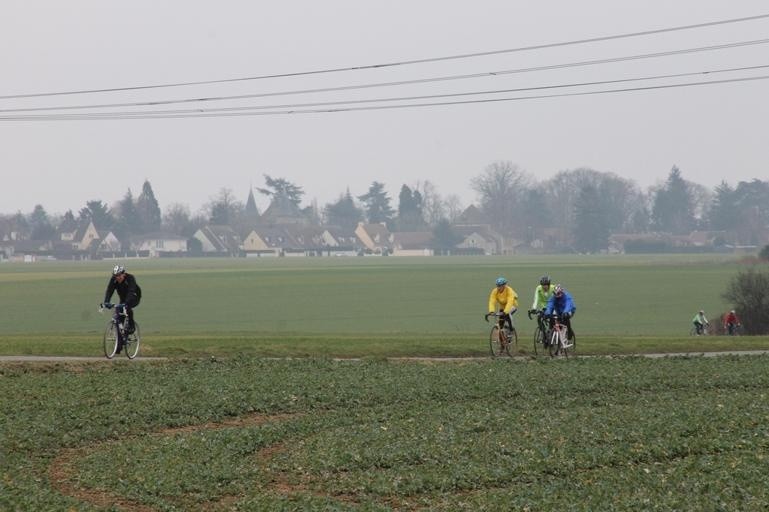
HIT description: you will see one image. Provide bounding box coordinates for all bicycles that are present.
[100,303,139,359]
[528,311,575,357]
[485,312,517,357]
[690,324,740,336]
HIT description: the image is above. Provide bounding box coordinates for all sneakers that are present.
[115,346,123,354]
[507,330,516,338]
[128,327,135,334]
[567,330,573,340]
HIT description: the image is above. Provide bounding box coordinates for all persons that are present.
[544,282,577,340]
[488,278,518,355]
[723,309,742,335]
[692,308,713,336]
[103,266,142,355]
[533,276,557,348]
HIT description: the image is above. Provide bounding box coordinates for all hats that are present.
[553,284,563,295]
[496,277,507,285]
[540,276,550,285]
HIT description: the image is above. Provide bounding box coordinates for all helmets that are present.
[700,310,704,314]
[112,265,125,276]
[731,310,735,314]
